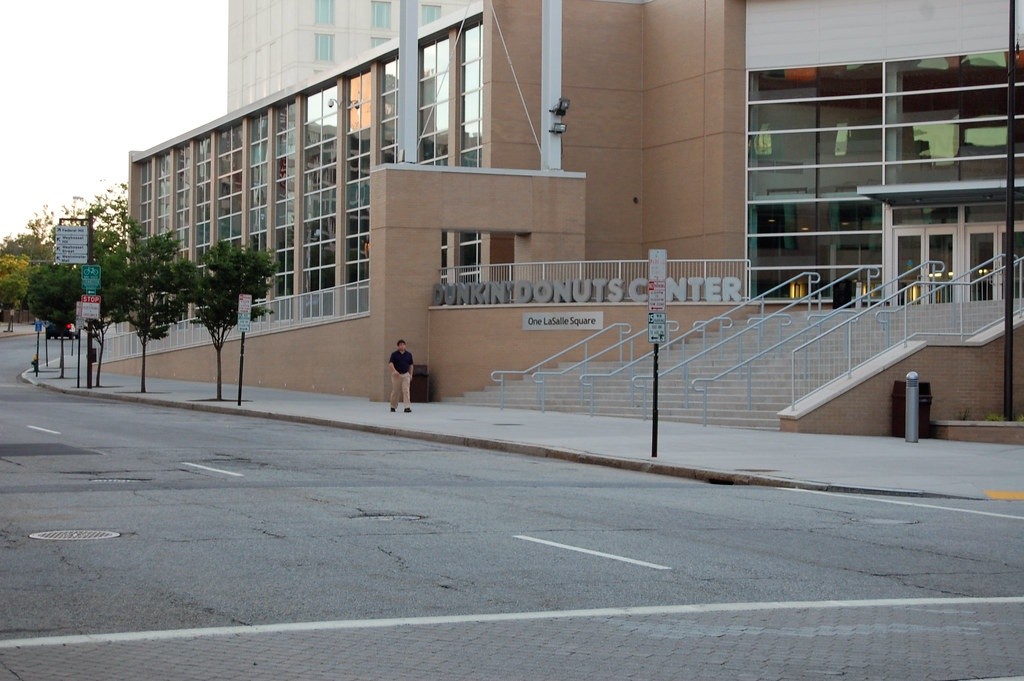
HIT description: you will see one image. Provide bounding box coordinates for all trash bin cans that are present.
[891,380,933,439]
[91,348,97,363]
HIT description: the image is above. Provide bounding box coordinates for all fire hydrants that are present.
[30,354,40,372]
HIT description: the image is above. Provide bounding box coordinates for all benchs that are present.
[767,187,807,196]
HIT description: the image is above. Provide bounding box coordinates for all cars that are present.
[45,321,79,340]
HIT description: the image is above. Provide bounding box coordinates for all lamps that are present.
[549,122,567,134]
[548,98,570,116]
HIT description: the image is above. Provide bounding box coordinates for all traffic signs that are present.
[647,280,666,342]
[238,294,252,332]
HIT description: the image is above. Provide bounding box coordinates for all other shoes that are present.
[391,407,396,412]
[404,408,411,412]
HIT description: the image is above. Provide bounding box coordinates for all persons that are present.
[388,340,413,412]
